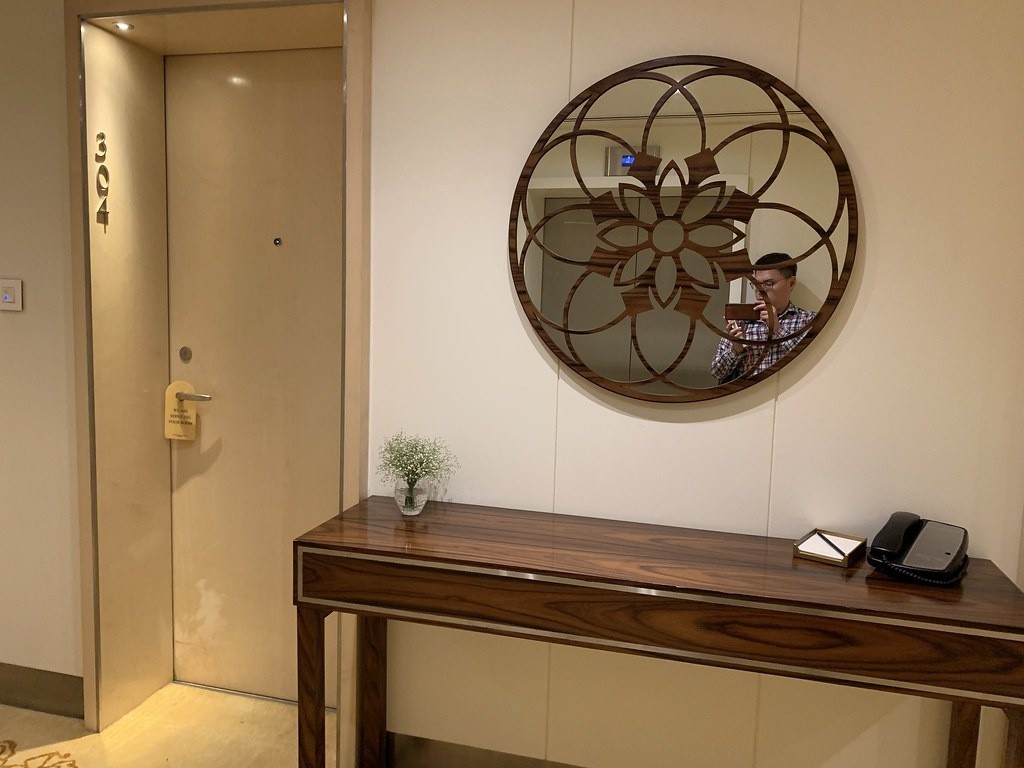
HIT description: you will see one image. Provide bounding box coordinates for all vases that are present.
[394,477,428,514]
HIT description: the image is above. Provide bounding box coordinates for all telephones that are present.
[866,511,969,588]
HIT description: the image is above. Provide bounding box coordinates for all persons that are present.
[710,253,816,384]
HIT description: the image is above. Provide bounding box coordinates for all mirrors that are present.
[505,52,860,403]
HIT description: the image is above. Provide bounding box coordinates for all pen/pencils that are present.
[816,531,846,557]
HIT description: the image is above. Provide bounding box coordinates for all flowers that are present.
[372,425,461,510]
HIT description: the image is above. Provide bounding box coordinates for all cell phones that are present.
[724,304,761,320]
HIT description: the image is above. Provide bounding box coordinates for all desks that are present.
[292,495,1024,768]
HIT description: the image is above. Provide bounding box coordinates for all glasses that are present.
[749,278,786,291]
